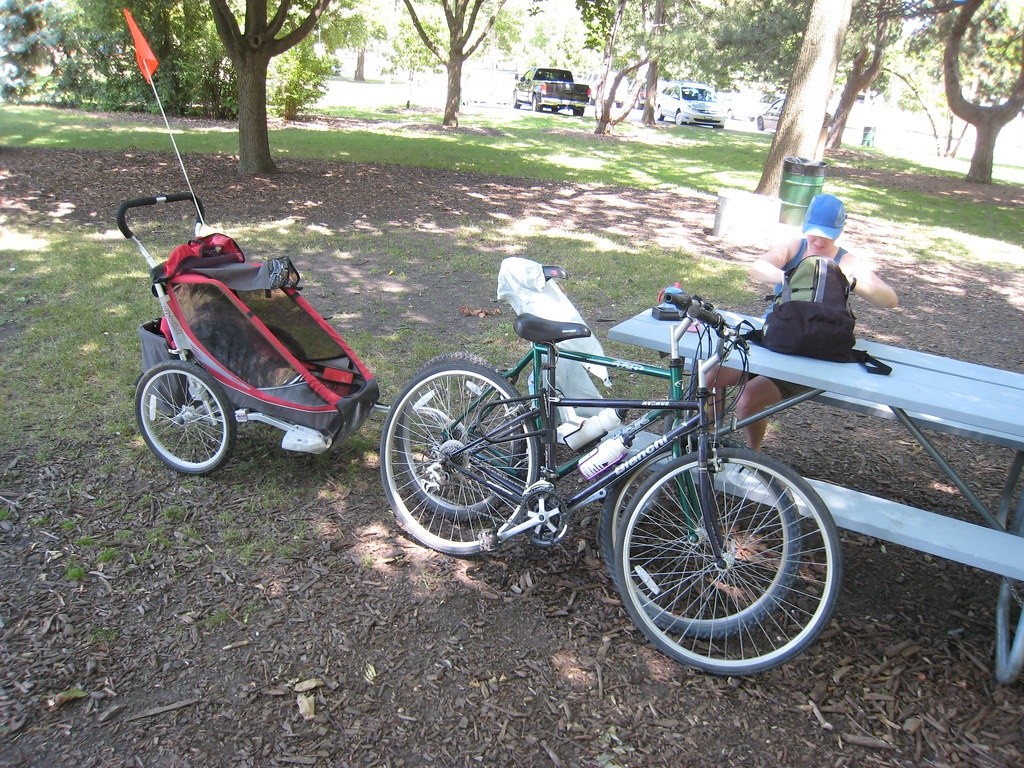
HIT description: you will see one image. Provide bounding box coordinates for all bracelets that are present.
[850,277,858,291]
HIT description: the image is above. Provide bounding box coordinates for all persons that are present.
[701,193,898,451]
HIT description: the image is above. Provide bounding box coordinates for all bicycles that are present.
[379,257,846,678]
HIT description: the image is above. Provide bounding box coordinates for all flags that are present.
[123,9,159,83]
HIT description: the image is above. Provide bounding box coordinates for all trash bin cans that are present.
[778,156,828,226]
[862,127,878,147]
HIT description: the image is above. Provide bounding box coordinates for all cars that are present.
[591,72,783,123]
[655,82,727,130]
[757,98,834,138]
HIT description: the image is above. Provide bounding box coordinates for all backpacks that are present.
[735,255,892,375]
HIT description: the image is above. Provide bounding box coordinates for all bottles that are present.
[578,433,635,482]
[564,408,630,452]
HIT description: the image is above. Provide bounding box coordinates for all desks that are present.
[607,299,1024,528]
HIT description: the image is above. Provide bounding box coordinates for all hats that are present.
[803,193,847,240]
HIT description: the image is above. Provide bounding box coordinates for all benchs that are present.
[598,388,1024,685]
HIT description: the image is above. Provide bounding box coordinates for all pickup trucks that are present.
[512,66,592,117]
